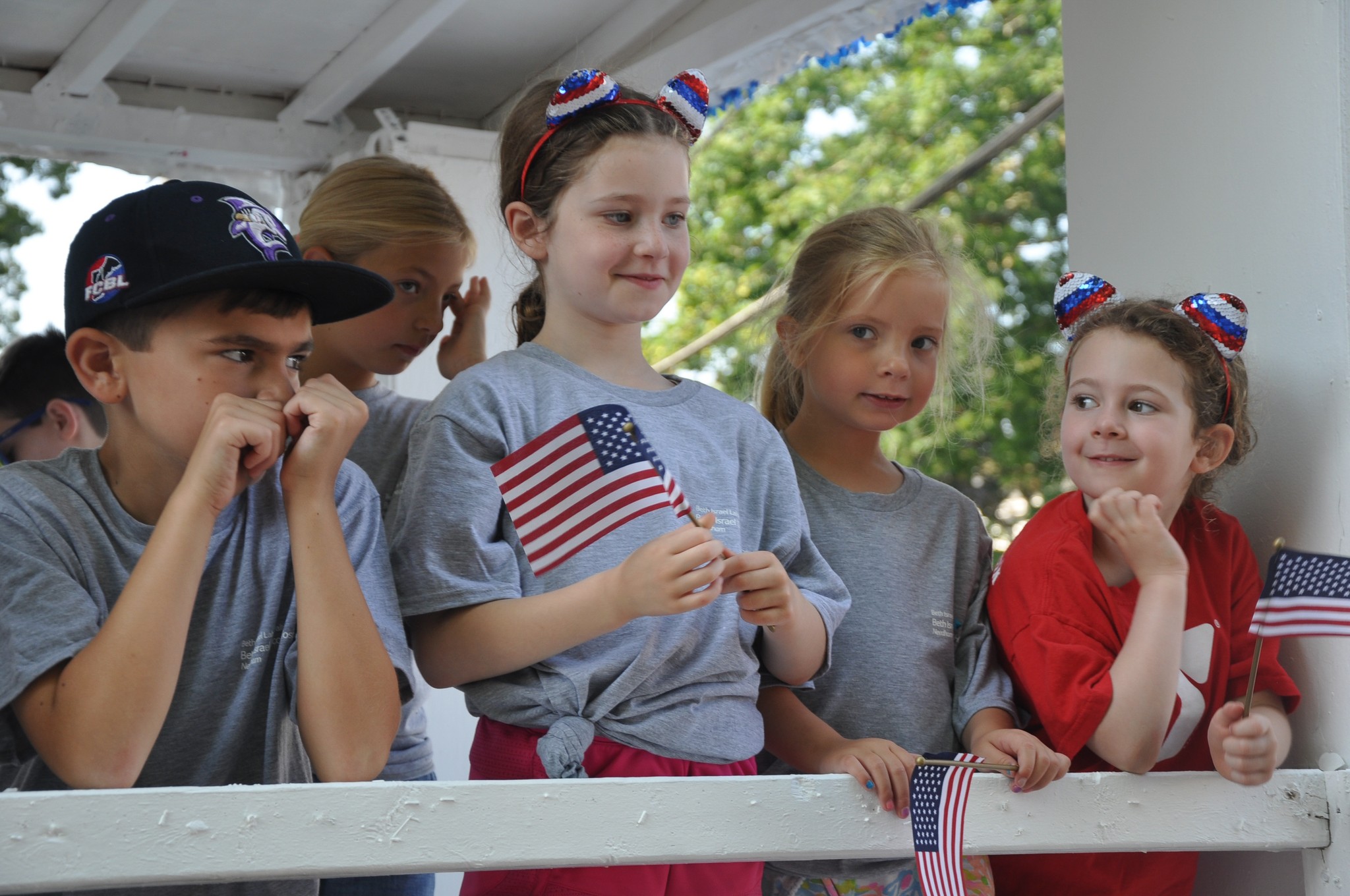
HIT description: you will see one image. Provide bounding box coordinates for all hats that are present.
[64,179,396,338]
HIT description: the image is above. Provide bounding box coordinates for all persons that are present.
[753,205,1072,896]
[387,66,851,896]
[0,174,423,896]
[0,327,110,466]
[984,270,1300,896]
[274,150,492,896]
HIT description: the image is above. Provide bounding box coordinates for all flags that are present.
[909,752,987,896]
[490,403,693,578]
[1248,549,1350,641]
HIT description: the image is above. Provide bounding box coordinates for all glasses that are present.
[0,408,47,465]
